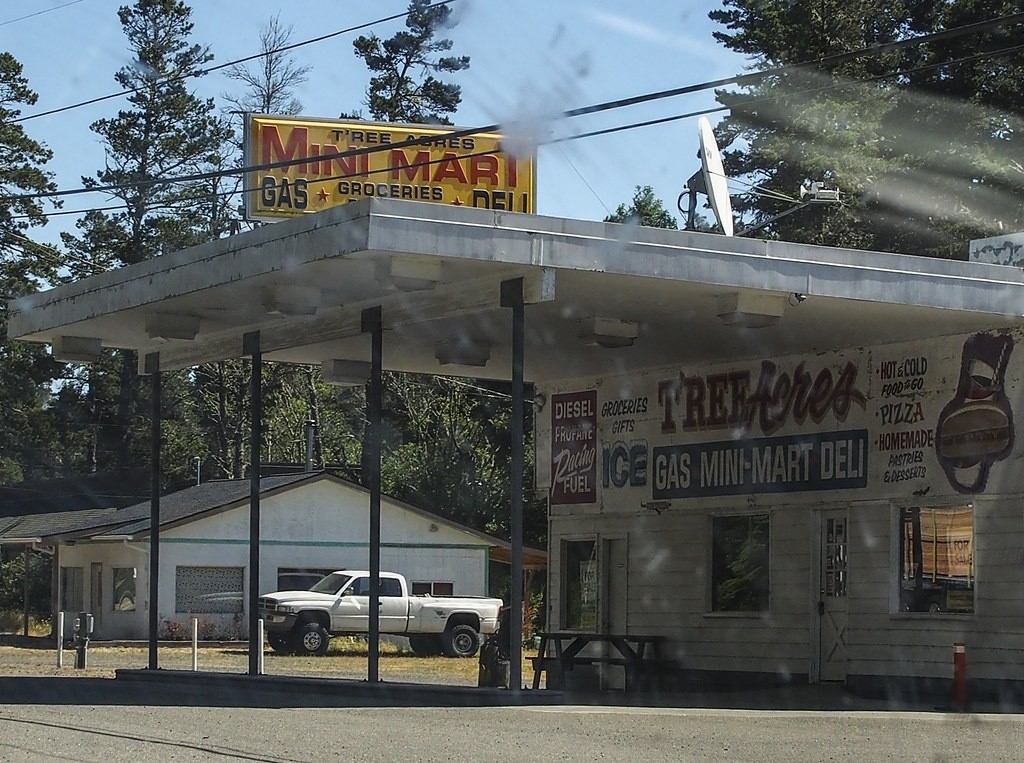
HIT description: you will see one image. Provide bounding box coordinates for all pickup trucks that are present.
[259,571,503,657]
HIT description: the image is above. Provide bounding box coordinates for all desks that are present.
[532,630,664,692]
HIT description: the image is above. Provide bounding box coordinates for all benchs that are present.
[525,657,606,689]
